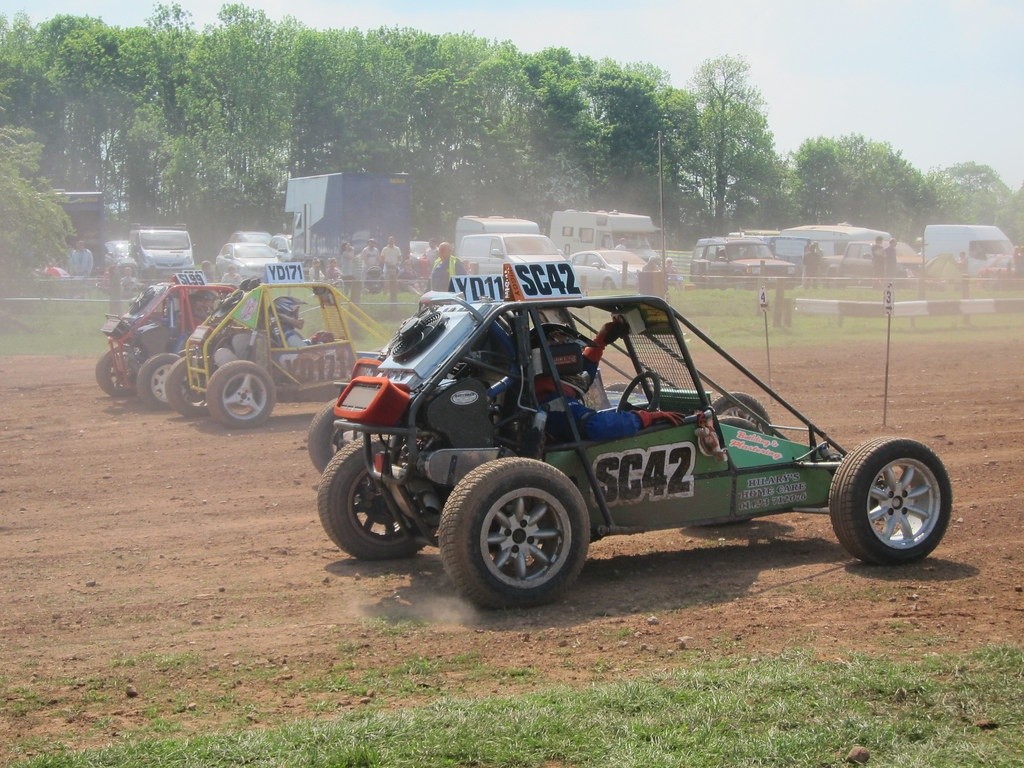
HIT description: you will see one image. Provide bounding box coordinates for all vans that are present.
[454,216,570,273]
[923,225,1018,275]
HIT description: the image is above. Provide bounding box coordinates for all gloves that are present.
[584,321,622,365]
[632,406,686,430]
[310,332,332,344]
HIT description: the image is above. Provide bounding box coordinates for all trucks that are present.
[550,208,664,262]
[129,222,195,278]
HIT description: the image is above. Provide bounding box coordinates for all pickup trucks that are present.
[820,240,927,277]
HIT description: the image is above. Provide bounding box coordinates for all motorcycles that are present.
[96,250,138,293]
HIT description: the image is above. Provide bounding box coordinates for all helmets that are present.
[270,297,309,330]
[531,323,596,394]
[189,291,218,320]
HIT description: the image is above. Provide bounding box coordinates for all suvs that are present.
[689,237,798,290]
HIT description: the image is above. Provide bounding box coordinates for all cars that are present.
[567,250,649,289]
[406,240,435,261]
[215,231,292,283]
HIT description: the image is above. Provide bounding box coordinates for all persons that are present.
[309,236,403,303]
[71,241,94,293]
[268,297,334,364]
[872,236,897,278]
[517,321,685,443]
[665,257,683,292]
[958,252,965,263]
[190,291,218,331]
[614,238,625,250]
[202,260,214,284]
[802,238,830,290]
[1014,246,1024,267]
[120,267,136,293]
[221,265,242,283]
[431,241,469,292]
